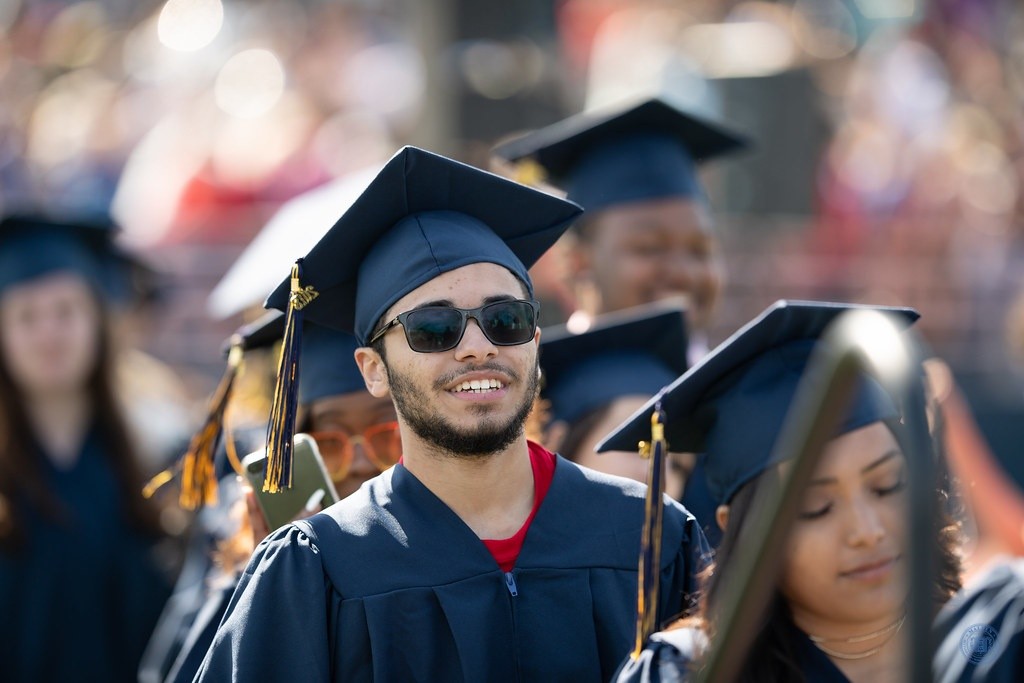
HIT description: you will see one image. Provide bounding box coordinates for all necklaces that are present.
[801,615,905,644]
[813,617,906,661]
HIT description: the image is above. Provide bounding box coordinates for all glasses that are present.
[308,420,403,484]
[368,299,539,353]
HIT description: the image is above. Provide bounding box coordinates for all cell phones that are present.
[243,431,338,534]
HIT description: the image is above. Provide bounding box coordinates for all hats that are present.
[260,146,586,493]
[492,97,754,221]
[537,297,690,428]
[594,299,923,660]
[140,307,368,511]
[0,210,122,293]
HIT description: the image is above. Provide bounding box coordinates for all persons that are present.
[185,144,713,683]
[0,101,1022,683]
